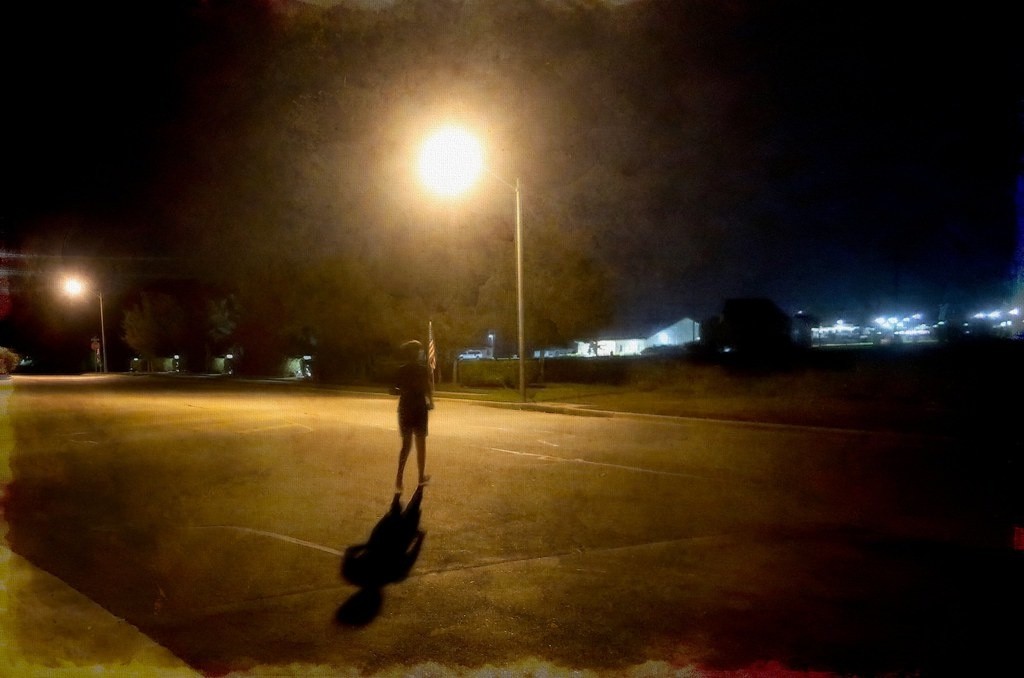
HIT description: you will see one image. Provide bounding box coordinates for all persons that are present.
[388,340,434,489]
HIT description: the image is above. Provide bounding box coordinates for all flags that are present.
[427,320,436,372]
